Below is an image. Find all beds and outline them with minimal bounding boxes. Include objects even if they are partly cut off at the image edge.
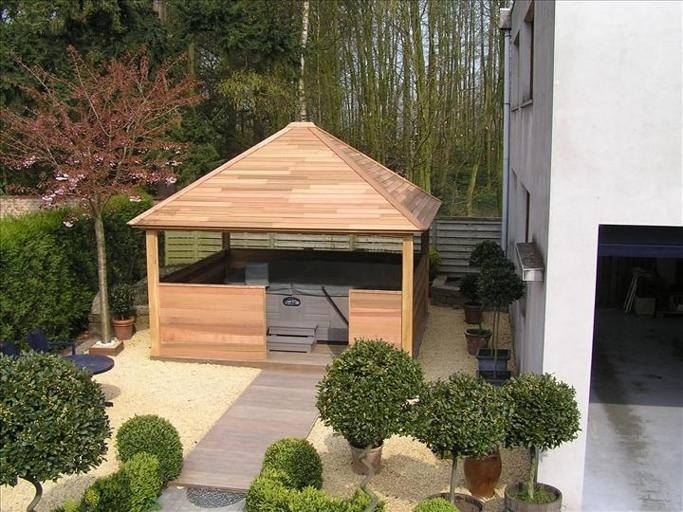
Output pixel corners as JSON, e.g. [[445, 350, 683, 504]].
[[225, 268, 361, 342]]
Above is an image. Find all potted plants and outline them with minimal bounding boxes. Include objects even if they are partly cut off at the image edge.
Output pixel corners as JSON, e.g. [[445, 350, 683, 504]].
[[405, 370, 583, 512], [107, 282, 136, 340], [316, 337, 427, 474], [458, 237, 529, 387]]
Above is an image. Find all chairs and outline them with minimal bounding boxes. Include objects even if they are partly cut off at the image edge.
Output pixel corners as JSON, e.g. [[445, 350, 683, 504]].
[[0, 341, 19, 356], [27, 328, 114, 407]]
